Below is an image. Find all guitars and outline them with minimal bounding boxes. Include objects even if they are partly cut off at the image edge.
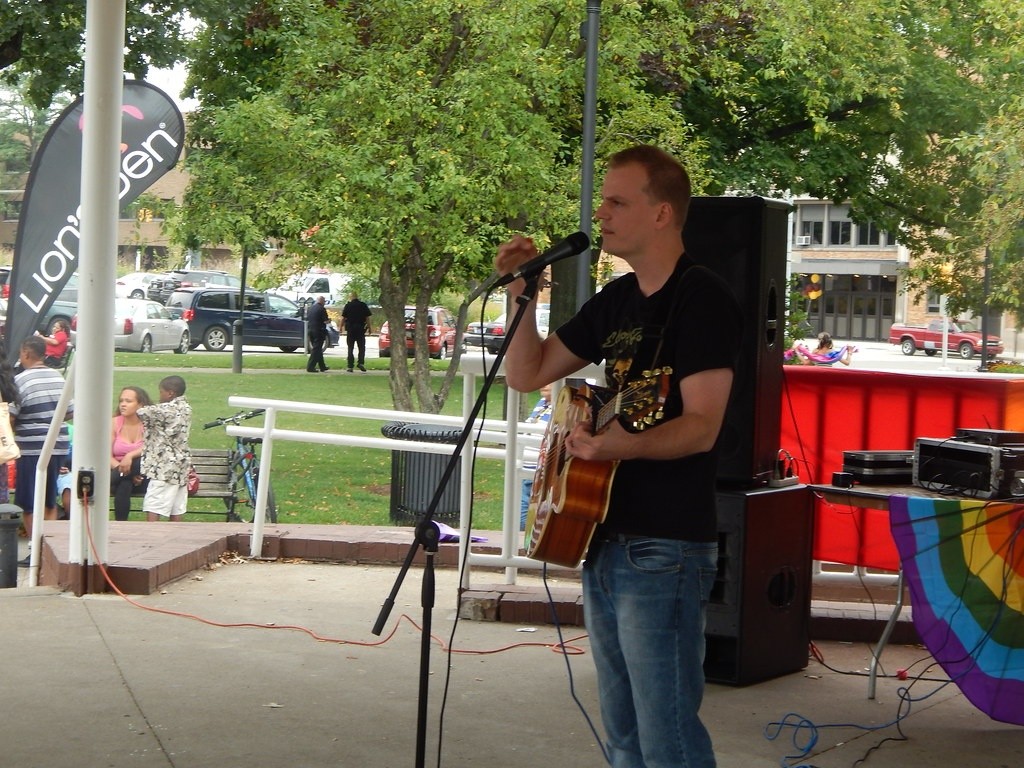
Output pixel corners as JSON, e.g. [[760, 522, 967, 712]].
[[525, 364, 675, 570]]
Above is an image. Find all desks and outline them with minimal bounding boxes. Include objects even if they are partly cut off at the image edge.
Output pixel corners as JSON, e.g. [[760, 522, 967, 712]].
[[807, 480, 1024, 699]]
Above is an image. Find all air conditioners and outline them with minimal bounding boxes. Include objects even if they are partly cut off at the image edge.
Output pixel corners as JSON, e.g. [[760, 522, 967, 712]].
[[796, 235, 810, 246]]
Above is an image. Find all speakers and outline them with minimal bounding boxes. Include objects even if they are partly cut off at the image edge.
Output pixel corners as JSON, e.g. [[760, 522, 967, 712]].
[[701, 483, 815, 689], [681, 196, 794, 491]]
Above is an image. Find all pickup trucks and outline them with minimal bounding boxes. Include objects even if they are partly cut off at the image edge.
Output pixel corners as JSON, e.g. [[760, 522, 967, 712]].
[[893, 315, 1004, 359]]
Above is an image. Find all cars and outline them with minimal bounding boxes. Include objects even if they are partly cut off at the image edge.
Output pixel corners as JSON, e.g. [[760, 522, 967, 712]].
[[166, 288, 339, 352], [467, 303, 549, 354], [116, 272, 167, 299], [69, 298, 192, 354]]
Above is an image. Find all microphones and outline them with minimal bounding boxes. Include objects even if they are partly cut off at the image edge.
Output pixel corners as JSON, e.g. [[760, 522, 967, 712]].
[[494, 232, 590, 288]]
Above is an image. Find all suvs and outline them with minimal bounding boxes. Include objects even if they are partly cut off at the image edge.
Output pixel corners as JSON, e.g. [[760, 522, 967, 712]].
[[379, 305, 467, 360], [147, 270, 257, 305], [0, 266, 78, 338]]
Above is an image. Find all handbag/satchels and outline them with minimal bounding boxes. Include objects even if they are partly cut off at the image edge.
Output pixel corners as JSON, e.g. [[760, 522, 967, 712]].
[[0, 398, 22, 465], [186, 466, 199, 493]]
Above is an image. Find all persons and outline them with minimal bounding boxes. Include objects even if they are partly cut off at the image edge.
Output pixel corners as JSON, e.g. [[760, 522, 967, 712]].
[[111, 386, 152, 520], [34, 321, 70, 366], [520, 384, 553, 532], [307, 296, 331, 372], [8, 335, 74, 567], [496, 146, 745, 768], [136, 375, 192, 522], [0, 336, 21, 500], [57, 424, 73, 519], [339, 292, 371, 372], [793, 332, 852, 367]]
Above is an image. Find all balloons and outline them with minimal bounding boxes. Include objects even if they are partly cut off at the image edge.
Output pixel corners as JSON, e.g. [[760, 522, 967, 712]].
[[801, 275, 822, 300]]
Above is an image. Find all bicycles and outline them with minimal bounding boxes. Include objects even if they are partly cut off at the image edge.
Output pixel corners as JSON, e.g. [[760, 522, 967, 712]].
[[202, 409, 277, 524]]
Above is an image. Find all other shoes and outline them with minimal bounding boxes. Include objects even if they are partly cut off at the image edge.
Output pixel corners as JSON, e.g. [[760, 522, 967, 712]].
[[308, 369, 318, 372], [347, 368, 352, 371], [357, 364, 366, 372], [322, 367, 329, 371], [17, 554, 29, 567]]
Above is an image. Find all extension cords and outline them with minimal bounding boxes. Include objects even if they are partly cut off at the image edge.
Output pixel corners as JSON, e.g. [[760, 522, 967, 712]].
[[769, 474, 799, 487]]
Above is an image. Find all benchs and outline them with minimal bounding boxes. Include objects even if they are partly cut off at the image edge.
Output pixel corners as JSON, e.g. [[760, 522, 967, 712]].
[[8, 448, 238, 521]]
